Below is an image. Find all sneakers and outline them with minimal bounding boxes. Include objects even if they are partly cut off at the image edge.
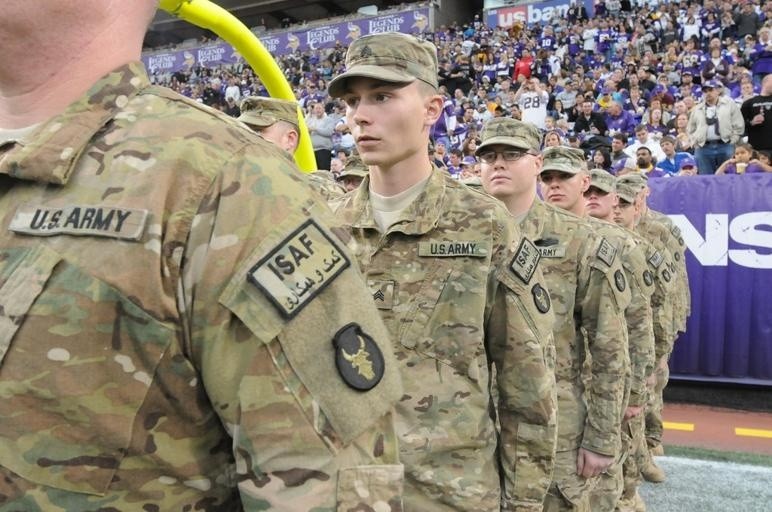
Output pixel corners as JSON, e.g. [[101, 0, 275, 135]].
[[640, 463, 665, 483], [634, 493, 646, 512], [651, 443, 666, 456]]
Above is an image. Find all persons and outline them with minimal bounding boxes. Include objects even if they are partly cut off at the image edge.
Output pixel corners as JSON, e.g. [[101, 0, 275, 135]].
[[1, 0, 772, 511]]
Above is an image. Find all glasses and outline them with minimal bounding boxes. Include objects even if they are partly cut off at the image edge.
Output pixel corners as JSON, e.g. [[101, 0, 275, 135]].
[[477, 147, 529, 164]]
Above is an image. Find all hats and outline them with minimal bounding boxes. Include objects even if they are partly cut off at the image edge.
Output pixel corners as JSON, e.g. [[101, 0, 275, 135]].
[[614, 173, 643, 192], [701, 79, 719, 91], [539, 145, 589, 179], [680, 158, 696, 170], [583, 168, 618, 193], [629, 171, 649, 186], [473, 116, 544, 158], [615, 182, 638, 204], [326, 30, 440, 99], [436, 139, 446, 145], [234, 94, 300, 130]]
